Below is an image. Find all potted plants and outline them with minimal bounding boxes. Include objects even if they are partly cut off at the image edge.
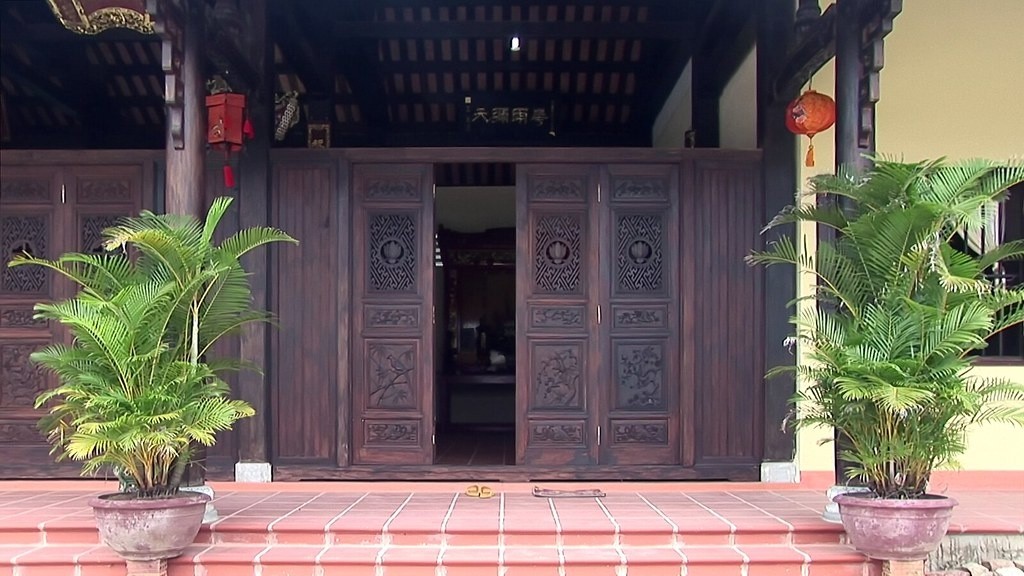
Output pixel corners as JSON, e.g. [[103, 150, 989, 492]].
[[745, 155, 1024, 562], [9, 197, 297, 560]]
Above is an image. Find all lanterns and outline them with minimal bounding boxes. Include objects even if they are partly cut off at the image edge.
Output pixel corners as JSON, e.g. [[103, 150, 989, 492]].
[[786, 86, 836, 167], [204, 95, 245, 189]]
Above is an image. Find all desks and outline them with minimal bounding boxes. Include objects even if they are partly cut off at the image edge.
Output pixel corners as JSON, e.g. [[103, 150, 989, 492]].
[[438, 372, 515, 436]]
